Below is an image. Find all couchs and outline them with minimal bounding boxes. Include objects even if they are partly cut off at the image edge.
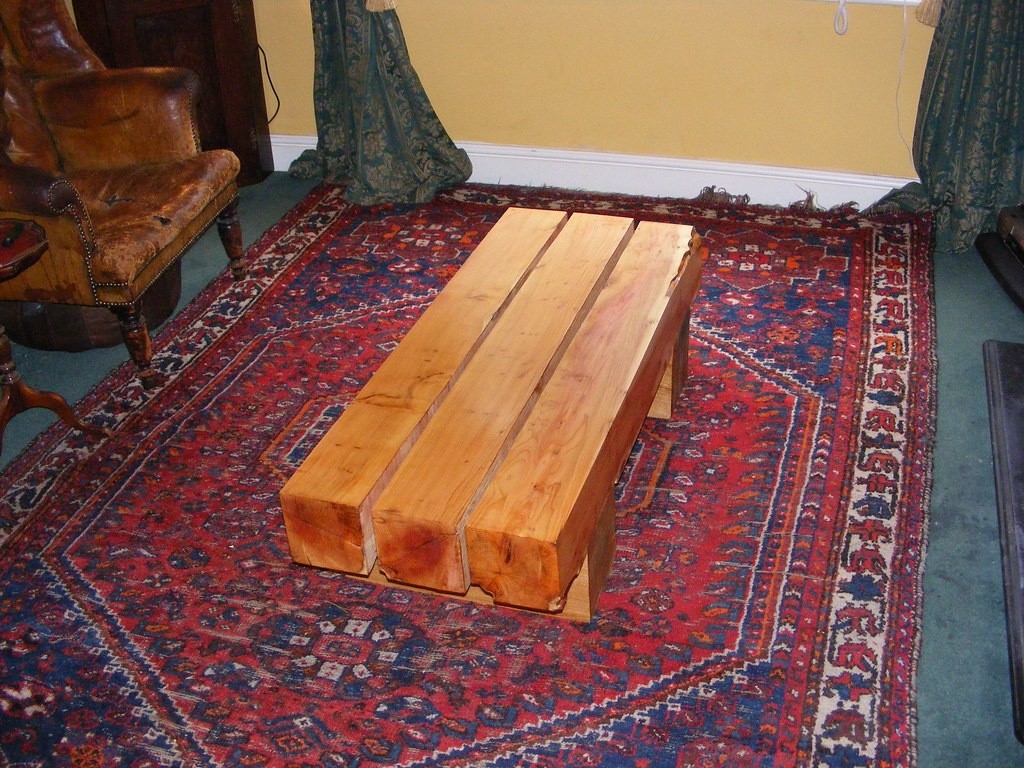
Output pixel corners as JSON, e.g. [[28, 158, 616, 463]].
[[0, 0, 247, 392]]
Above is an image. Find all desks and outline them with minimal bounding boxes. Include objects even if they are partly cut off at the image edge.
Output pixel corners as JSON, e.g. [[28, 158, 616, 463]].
[[280, 206, 703, 624], [0, 218, 115, 455]]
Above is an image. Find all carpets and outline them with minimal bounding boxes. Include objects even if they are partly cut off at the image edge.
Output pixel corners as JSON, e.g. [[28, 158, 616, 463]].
[[0, 183, 938, 767]]
